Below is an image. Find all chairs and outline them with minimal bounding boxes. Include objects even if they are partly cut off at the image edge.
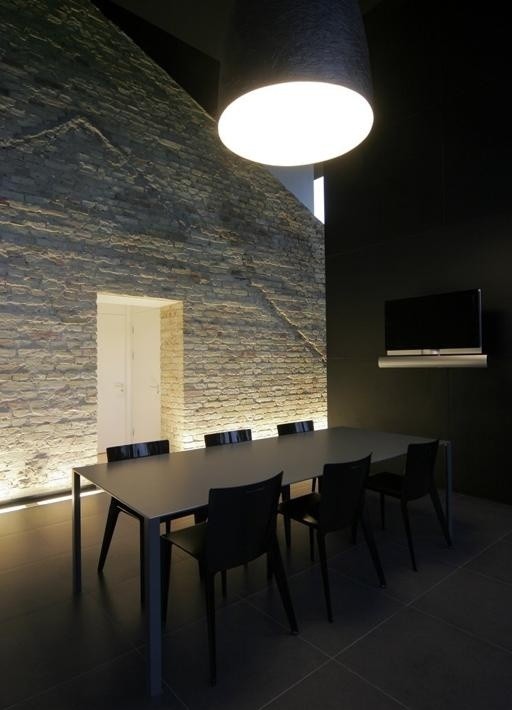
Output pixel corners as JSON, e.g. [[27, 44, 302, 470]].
[[277, 421, 316, 502], [266, 452, 388, 625], [192, 429, 251, 525], [349, 440, 455, 572], [97, 440, 171, 611], [161, 470, 298, 688]]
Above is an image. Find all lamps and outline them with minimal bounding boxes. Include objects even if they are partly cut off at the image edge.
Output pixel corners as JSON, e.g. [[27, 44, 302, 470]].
[[215, 0, 378, 167]]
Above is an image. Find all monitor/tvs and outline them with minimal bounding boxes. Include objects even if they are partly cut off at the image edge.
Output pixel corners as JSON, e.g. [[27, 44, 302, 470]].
[[385, 289, 482, 357]]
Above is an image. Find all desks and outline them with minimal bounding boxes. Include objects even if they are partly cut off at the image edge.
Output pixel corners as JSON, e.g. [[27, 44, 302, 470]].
[[71, 427, 454, 699]]
